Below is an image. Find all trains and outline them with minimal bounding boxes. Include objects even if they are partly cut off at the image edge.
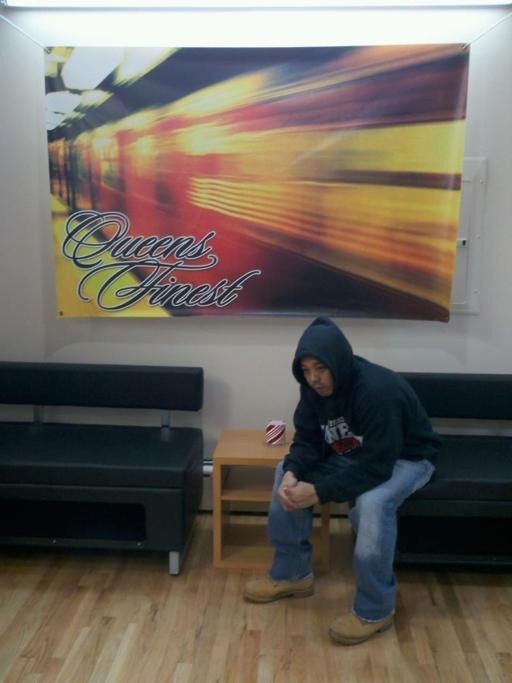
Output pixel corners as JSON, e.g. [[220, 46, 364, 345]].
[[49, 47, 466, 320]]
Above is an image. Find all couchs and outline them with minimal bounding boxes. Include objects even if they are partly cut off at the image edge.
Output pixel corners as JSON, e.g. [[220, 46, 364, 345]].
[[0, 360, 203, 575], [352, 373, 511, 573]]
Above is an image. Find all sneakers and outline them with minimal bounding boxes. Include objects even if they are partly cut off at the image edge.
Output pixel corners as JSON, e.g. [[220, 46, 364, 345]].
[[243, 571, 315, 604], [329, 606, 395, 646]]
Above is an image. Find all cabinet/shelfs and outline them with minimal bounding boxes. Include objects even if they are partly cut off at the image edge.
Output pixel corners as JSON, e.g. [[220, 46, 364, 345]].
[[212, 428, 330, 573]]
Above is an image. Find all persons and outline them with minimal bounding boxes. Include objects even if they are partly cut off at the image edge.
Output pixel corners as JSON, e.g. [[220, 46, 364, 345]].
[[242, 316, 436, 645]]
